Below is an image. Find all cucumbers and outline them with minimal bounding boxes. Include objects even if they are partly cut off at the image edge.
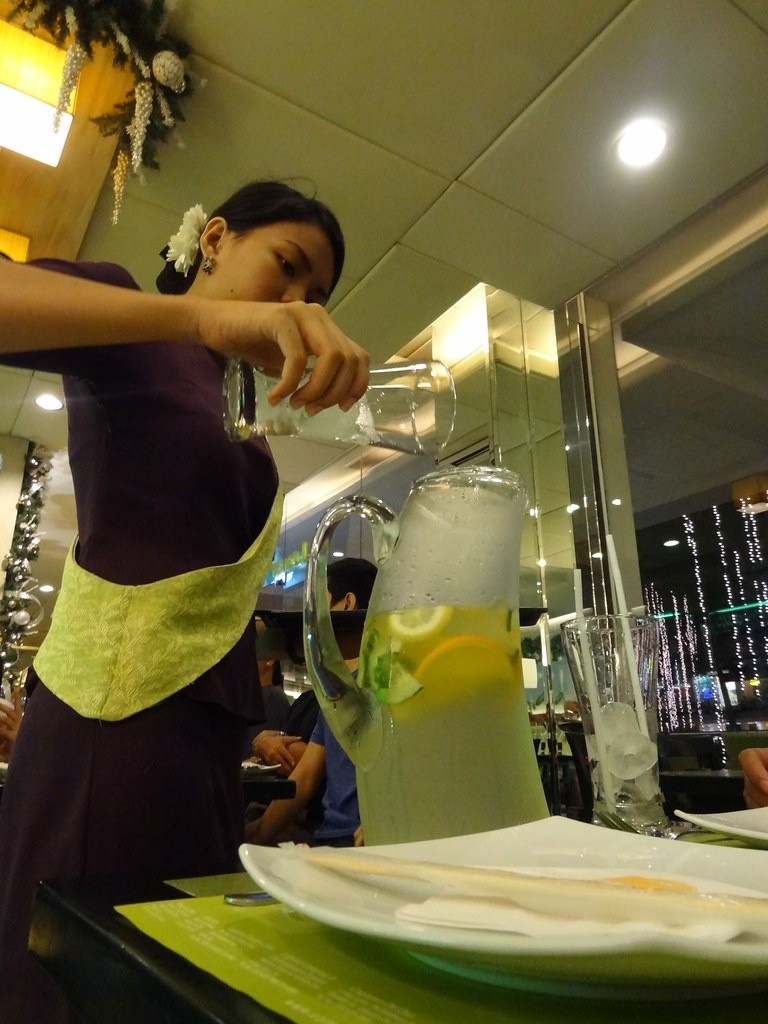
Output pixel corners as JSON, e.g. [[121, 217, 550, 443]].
[[355, 629, 423, 706]]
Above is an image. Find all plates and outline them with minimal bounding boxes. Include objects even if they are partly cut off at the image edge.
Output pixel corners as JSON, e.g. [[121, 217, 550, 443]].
[[242, 762, 282, 775], [239, 815, 767, 1004], [674, 807, 768, 848]]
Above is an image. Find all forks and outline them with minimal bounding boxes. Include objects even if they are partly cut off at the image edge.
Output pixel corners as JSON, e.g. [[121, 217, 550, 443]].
[[594, 811, 715, 841]]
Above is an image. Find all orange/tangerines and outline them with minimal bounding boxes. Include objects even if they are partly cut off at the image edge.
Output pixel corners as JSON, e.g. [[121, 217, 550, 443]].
[[415, 635, 512, 701]]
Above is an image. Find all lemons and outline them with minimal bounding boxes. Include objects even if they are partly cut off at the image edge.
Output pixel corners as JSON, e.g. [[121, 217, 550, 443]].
[[388, 604, 453, 640]]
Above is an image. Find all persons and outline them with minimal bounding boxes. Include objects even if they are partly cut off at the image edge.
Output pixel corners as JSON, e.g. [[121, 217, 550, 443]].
[[0, 177, 372, 1022], [244, 557, 378, 847], [738, 747, 768, 810]]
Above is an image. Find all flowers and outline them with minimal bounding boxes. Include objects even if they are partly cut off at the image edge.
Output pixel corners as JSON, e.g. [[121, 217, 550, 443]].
[[165, 203, 207, 279]]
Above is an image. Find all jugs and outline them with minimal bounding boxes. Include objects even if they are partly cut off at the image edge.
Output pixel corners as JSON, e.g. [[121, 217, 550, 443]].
[[303, 466, 550, 846]]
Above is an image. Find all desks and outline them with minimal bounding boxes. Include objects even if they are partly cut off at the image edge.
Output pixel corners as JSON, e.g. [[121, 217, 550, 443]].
[[35, 812, 768, 1024]]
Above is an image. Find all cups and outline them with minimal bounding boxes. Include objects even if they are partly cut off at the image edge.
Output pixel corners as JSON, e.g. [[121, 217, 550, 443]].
[[222, 354, 457, 456], [560, 615, 673, 841]]
